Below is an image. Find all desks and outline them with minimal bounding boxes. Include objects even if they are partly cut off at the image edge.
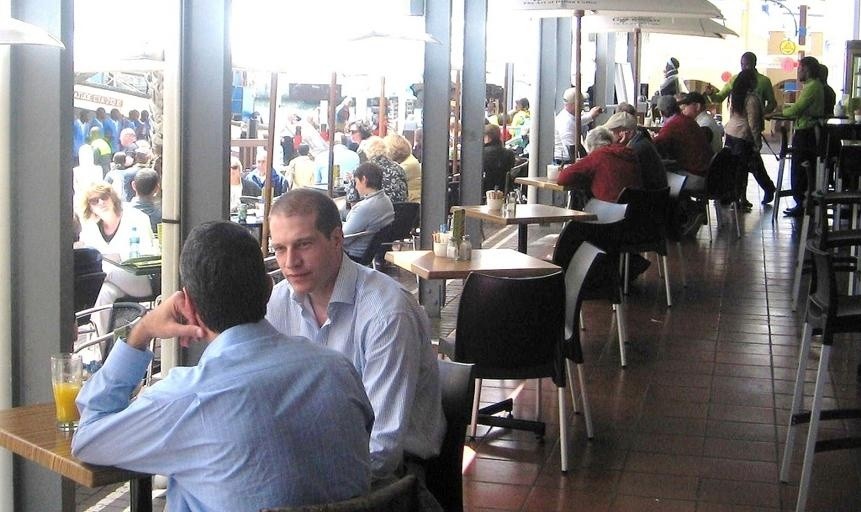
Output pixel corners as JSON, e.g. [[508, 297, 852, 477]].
[[515, 178, 573, 263]]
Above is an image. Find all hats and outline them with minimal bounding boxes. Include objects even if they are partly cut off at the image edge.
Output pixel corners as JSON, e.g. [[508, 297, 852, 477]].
[[563, 88, 590, 103], [653, 95, 676, 110], [676, 92, 705, 106], [602, 112, 637, 129]]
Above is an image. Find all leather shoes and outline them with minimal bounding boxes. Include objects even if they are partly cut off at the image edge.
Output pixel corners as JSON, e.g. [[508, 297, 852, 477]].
[[740, 187, 802, 215]]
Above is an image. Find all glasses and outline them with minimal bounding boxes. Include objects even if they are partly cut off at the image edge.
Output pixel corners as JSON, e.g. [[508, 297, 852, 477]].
[[88, 191, 110, 205]]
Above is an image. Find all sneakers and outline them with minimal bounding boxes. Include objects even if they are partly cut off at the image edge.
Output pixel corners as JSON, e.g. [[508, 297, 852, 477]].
[[629, 256, 651, 280]]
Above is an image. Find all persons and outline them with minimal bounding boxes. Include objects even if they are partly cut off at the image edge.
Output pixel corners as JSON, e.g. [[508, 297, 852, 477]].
[[70, 217, 375, 512], [264, 186, 450, 483], [67, 96, 534, 366], [552, 50, 838, 289]]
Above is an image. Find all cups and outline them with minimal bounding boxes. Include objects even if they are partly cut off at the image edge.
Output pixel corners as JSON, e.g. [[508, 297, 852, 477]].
[[547, 165, 558, 180], [238, 204, 248, 221], [50, 352, 83, 431], [336, 178, 344, 187], [255, 203, 264, 217], [487, 199, 503, 210], [644, 118, 651, 127], [433, 242, 447, 257], [834, 105, 846, 118]]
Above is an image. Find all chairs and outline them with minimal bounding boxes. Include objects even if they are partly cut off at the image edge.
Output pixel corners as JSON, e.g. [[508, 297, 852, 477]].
[[445, 182, 462, 228], [686, 149, 742, 245], [581, 198, 630, 223], [619, 171, 687, 307], [768, 112, 861, 512], [502, 158, 529, 197], [714, 143, 746, 240]]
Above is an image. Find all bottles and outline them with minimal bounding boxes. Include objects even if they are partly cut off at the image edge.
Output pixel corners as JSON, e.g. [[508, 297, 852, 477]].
[[854, 109, 861, 122], [655, 118, 660, 127], [459, 235, 472, 260], [509, 192, 517, 210], [128, 227, 140, 258], [447, 237, 459, 260]]
